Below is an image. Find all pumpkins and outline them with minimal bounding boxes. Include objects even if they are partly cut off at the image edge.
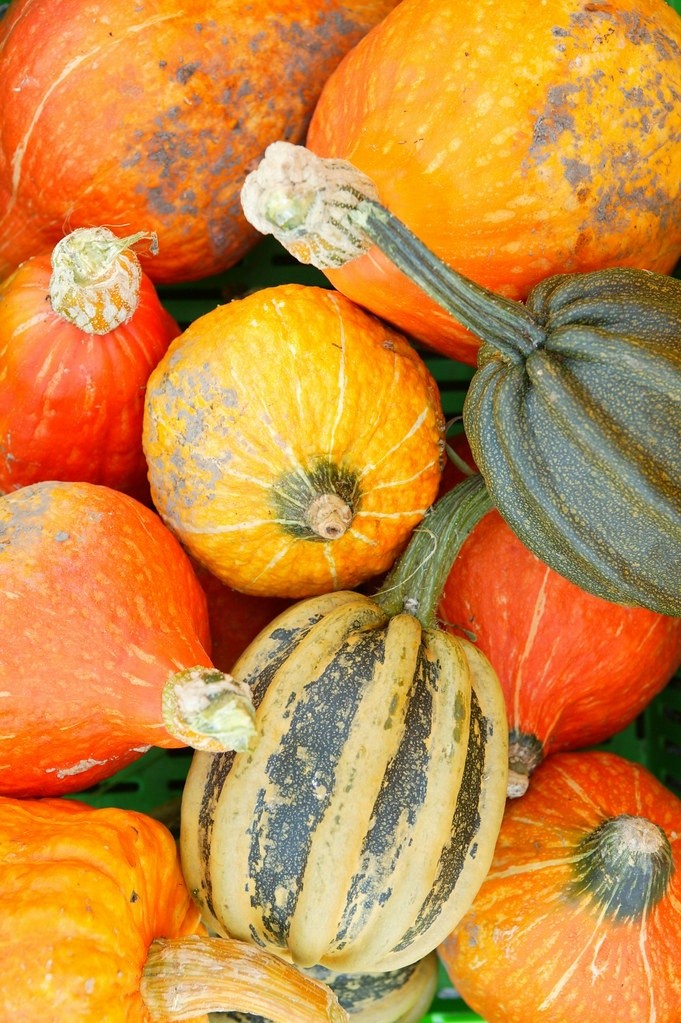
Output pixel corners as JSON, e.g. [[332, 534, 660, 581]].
[[0, 0, 681, 1023]]
